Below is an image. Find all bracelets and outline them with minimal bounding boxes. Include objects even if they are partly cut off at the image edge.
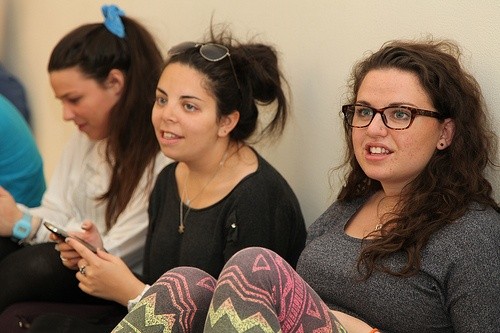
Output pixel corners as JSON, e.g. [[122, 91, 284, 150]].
[[369, 328, 378, 333]]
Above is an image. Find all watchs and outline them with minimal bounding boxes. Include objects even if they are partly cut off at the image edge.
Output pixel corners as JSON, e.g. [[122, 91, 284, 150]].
[[12, 213, 33, 242]]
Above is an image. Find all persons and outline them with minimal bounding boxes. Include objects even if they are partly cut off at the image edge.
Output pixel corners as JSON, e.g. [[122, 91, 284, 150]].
[[0, 5, 305, 333], [109, 40, 500, 333]]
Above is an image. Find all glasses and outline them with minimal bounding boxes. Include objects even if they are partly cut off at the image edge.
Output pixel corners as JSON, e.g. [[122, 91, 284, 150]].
[[167, 40, 240, 87], [342, 103, 443, 130]]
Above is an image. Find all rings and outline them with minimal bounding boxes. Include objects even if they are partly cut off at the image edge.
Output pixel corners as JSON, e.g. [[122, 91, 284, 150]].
[[78, 265, 86, 274]]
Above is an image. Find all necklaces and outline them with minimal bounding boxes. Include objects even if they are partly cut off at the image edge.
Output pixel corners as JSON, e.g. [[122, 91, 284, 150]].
[[177, 151, 229, 235], [374, 192, 400, 231]]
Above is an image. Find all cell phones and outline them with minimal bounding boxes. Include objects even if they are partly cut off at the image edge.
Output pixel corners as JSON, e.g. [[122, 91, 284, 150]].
[[44, 222, 98, 255]]
